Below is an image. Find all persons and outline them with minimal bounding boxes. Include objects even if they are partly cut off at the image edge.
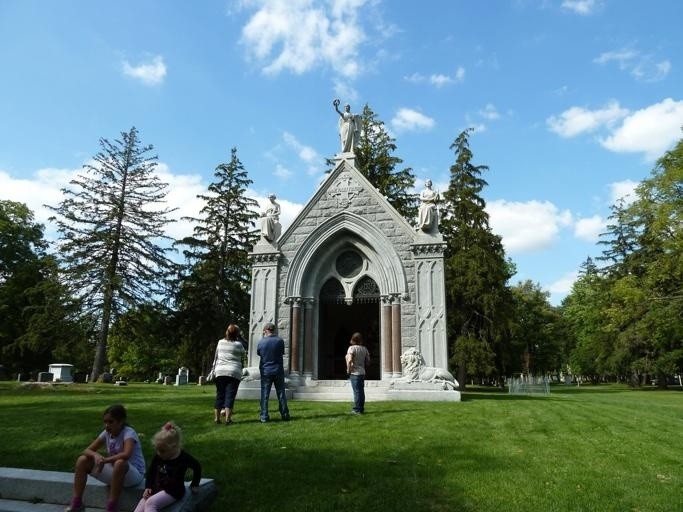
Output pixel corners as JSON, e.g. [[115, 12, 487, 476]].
[[332, 98, 363, 154], [63, 402, 145, 510], [416, 179, 441, 231], [255, 323, 290, 423], [211, 324, 247, 424], [259, 192, 282, 240], [128, 419, 201, 512], [343, 331, 373, 415]]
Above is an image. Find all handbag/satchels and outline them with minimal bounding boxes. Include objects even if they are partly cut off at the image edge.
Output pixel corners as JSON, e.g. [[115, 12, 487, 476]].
[[205, 372, 215, 381]]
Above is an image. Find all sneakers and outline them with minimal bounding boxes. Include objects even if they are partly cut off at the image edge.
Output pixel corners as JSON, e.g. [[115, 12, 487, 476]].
[[213, 409, 289, 426], [351, 409, 364, 416]]
